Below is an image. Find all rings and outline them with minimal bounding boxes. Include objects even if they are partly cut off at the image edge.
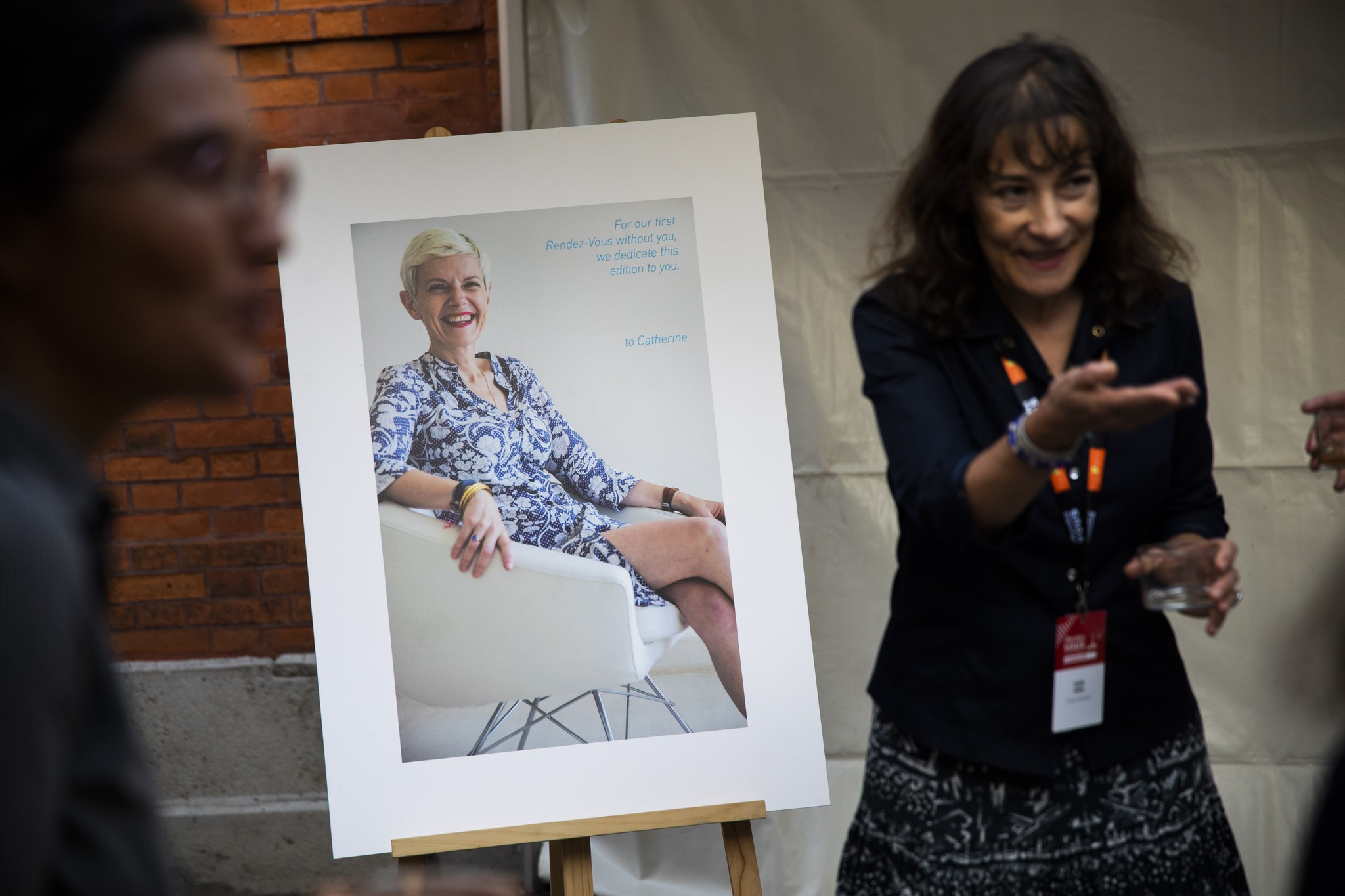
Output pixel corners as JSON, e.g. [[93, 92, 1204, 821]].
[[470, 535, 482, 542]]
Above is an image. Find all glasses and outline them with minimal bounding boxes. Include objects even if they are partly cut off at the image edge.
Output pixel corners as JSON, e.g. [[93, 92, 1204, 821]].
[[37, 131, 294, 218]]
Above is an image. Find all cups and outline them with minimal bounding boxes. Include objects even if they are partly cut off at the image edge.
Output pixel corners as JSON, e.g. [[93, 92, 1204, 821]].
[[1137, 540, 1218, 608]]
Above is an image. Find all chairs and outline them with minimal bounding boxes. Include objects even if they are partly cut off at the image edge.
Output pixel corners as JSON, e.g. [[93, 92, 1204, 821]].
[[379, 500, 691, 756]]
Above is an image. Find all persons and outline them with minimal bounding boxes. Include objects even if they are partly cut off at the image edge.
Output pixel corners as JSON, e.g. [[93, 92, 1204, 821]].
[[1291, 392, 1345, 896], [368, 228, 749, 722], [2, 0, 300, 896], [825, 40, 1254, 896]]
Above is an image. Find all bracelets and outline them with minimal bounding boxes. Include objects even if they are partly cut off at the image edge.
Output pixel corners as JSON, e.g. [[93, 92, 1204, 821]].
[[1007, 416, 1080, 468], [450, 478, 493, 519], [661, 487, 679, 512]]
